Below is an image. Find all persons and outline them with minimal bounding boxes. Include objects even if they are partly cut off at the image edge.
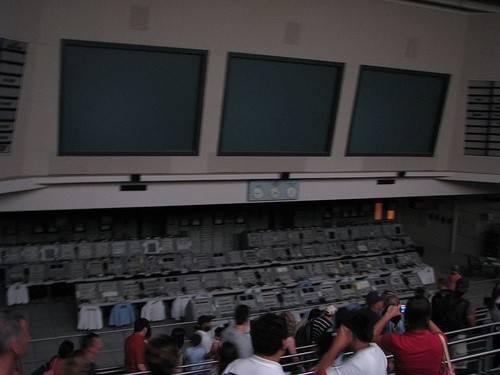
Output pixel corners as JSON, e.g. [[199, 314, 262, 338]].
[[0, 264, 500, 375]]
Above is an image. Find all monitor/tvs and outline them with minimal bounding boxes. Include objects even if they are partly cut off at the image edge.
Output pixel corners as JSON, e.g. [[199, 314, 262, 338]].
[[58, 38, 452, 157]]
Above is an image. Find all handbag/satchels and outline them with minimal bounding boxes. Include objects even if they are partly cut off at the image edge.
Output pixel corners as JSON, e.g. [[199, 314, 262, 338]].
[[437, 333, 455, 375]]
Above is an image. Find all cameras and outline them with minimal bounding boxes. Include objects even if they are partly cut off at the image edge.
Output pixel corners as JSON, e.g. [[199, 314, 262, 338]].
[[400, 305, 406, 313]]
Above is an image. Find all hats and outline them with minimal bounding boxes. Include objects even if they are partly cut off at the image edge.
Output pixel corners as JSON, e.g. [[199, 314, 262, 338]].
[[325, 305, 338, 316], [199, 314, 215, 326]]
